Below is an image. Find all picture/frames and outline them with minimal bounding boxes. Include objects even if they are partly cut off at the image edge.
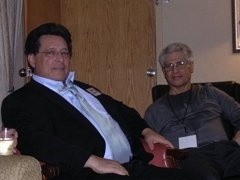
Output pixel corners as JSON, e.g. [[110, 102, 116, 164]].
[[231, 0, 240, 53]]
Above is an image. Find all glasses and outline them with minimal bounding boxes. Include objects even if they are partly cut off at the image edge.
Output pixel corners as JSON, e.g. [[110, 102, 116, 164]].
[[38, 49, 70, 58], [163, 60, 190, 70]]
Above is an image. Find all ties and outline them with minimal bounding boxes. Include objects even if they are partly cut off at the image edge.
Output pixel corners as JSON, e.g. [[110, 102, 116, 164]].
[[61, 84, 129, 164]]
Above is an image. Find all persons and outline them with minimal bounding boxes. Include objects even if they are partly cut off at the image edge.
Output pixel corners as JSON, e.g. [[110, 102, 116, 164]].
[[144, 43, 240, 180], [0, 22, 207, 180]]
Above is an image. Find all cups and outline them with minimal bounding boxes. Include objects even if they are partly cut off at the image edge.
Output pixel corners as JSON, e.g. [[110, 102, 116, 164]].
[[1, 127, 16, 156]]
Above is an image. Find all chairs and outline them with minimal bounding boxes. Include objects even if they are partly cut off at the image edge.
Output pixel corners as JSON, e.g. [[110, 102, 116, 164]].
[[142, 81, 240, 168]]
